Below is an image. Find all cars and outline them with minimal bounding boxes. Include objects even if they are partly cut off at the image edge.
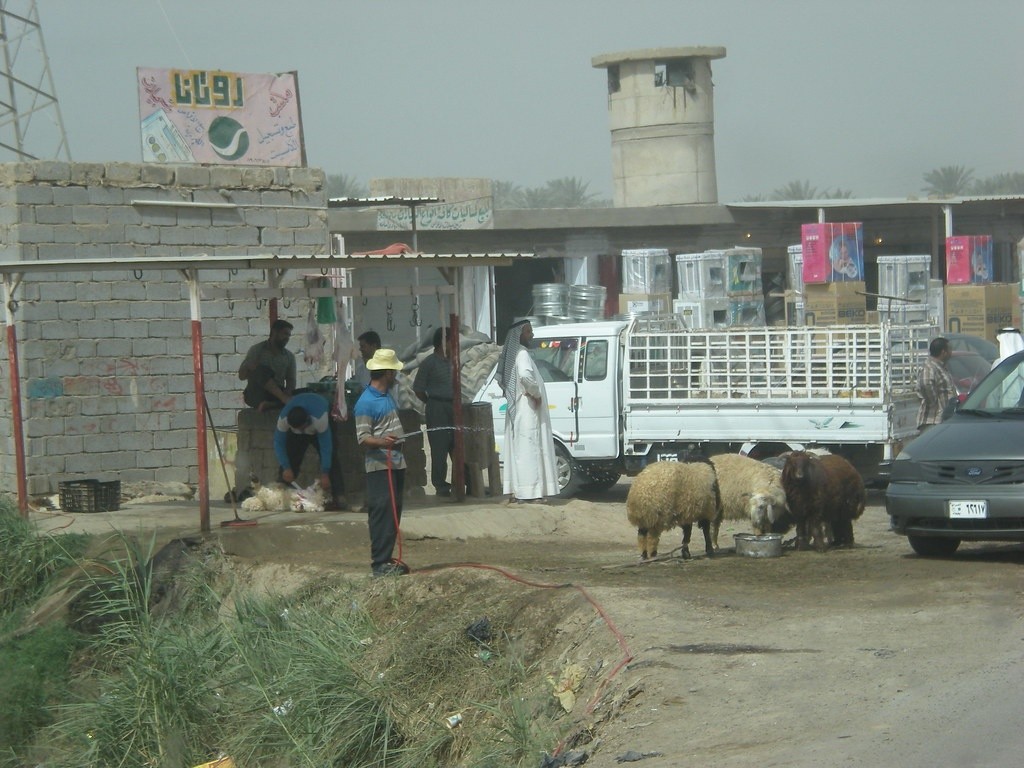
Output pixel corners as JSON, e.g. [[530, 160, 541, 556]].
[[796, 335, 998, 392], [884, 351, 1024, 558]]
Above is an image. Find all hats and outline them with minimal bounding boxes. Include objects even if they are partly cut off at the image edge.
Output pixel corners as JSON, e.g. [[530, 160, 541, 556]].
[[366, 348, 403, 370]]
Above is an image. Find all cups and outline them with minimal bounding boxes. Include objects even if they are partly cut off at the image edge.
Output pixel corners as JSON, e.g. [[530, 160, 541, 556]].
[[445, 713, 463, 730]]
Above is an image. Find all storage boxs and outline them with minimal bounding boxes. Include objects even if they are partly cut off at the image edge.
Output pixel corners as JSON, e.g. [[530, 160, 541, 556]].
[[802, 221, 864, 284], [58, 479, 121, 513], [618, 292, 672, 318], [943, 284, 1013, 353], [804, 282, 865, 353], [946, 236, 993, 284]]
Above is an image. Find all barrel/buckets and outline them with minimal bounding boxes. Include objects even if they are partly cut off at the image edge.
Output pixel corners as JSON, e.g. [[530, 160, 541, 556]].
[[512, 282, 609, 328]]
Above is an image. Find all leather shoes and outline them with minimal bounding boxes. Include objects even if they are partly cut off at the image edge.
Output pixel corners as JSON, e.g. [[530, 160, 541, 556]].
[[372, 562, 407, 578]]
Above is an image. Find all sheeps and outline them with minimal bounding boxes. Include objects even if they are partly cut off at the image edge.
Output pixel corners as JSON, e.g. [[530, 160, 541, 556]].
[[709, 454, 794, 553], [777, 450, 867, 554], [624, 450, 722, 564]]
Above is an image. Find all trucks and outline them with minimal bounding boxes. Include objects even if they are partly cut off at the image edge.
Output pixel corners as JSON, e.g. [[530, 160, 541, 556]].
[[474, 312, 939, 502]]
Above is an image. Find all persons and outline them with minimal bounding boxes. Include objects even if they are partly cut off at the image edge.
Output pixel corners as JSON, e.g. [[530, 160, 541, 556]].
[[239, 320, 296, 408], [985, 327, 1024, 409], [835, 240, 853, 270], [977, 249, 986, 273], [915, 337, 958, 434], [273, 393, 348, 510], [495, 318, 560, 504], [413, 327, 490, 498], [354, 331, 411, 576]]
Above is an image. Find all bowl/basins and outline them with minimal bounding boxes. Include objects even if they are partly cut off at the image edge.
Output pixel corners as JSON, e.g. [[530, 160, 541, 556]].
[[733, 531, 786, 559]]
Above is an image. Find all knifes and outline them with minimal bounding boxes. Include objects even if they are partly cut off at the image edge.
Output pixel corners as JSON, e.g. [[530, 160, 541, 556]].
[[290, 480, 305, 490]]
